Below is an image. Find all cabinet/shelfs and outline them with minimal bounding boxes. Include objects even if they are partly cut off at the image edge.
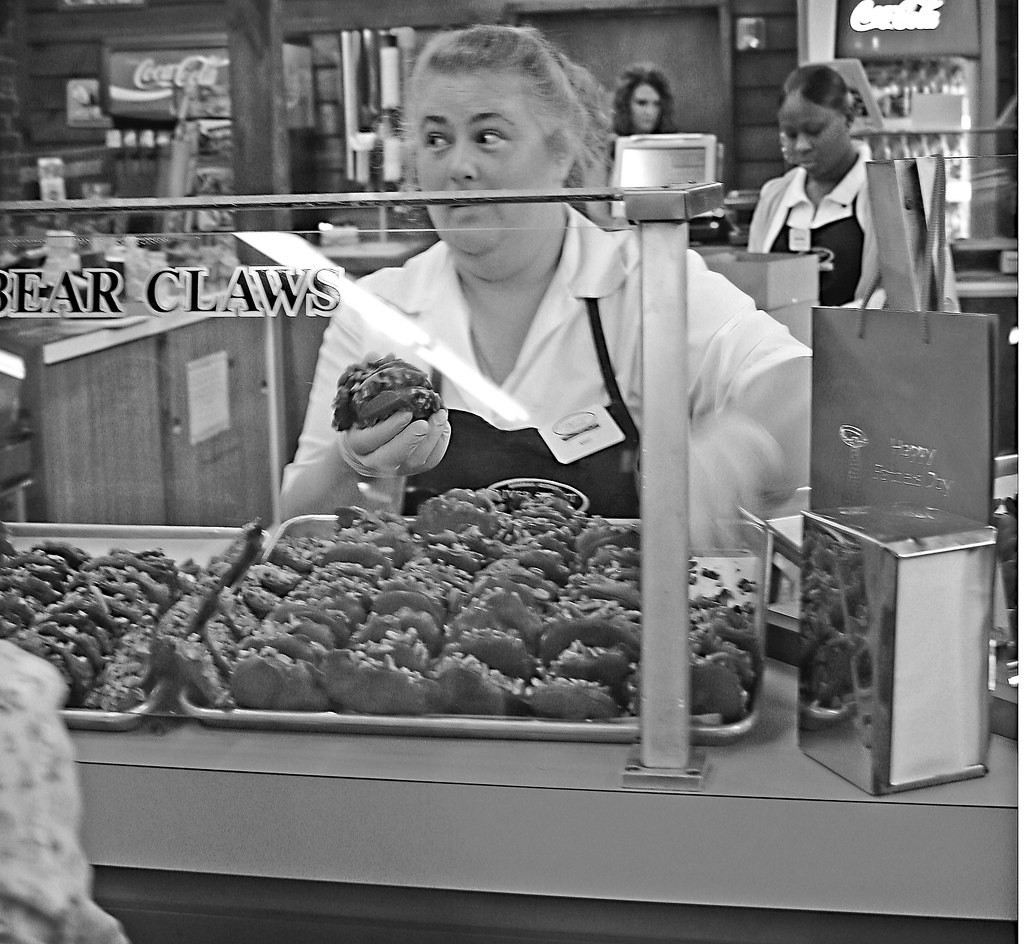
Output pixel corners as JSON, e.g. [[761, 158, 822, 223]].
[[0, 305, 332, 530]]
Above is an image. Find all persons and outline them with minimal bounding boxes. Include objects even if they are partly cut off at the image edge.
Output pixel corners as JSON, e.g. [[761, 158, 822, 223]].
[[747, 67, 893, 311], [276, 23, 813, 554], [579, 61, 678, 231]]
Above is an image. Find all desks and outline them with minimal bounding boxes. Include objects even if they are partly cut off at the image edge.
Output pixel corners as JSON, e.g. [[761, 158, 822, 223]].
[[320, 240, 431, 269]]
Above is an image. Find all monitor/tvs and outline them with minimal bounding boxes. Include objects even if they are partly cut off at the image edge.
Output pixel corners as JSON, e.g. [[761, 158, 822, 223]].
[[610, 132, 717, 217]]
